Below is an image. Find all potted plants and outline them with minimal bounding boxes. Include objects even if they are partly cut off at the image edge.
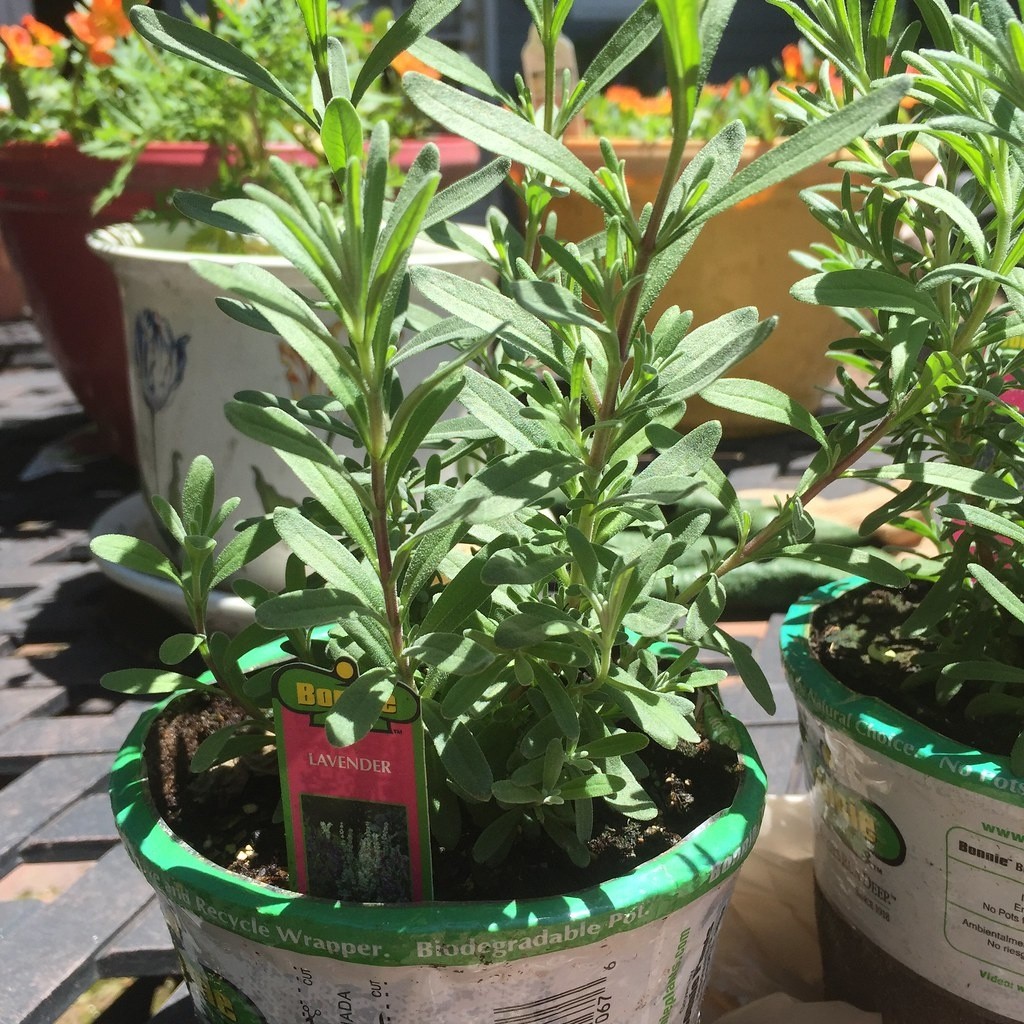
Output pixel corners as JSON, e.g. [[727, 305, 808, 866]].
[[87, 1, 1023, 1022]]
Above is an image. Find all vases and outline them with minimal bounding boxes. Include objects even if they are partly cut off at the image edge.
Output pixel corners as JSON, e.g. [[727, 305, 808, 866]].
[[509, 138, 945, 442], [1, 126, 479, 481]]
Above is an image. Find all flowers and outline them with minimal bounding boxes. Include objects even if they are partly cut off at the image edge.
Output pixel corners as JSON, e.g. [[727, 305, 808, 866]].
[[0, 0, 448, 143], [558, 45, 928, 139]]
